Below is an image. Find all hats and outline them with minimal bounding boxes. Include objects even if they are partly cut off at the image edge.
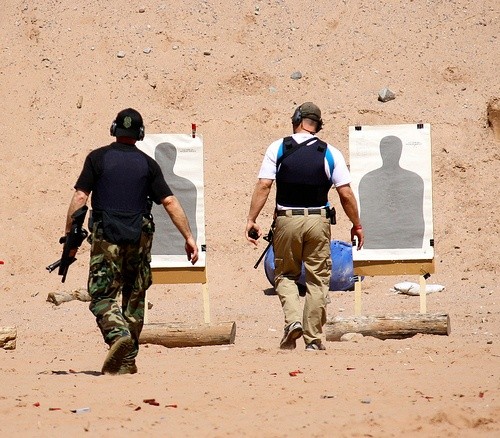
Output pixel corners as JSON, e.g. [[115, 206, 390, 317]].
[[292, 101, 322, 121], [112, 107, 144, 139]]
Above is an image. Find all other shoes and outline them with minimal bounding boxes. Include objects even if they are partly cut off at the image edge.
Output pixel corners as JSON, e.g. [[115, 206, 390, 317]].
[[279, 322, 304, 350], [119, 364, 139, 374], [101, 335, 134, 376], [305, 340, 329, 352]]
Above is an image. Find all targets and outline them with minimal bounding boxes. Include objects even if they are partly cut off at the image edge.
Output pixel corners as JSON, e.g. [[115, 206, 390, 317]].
[[133, 132, 206, 268], [348, 121, 434, 261]]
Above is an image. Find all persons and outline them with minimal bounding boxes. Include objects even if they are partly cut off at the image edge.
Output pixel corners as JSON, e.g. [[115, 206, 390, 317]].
[[245, 101, 364, 352], [64, 107, 198, 375]]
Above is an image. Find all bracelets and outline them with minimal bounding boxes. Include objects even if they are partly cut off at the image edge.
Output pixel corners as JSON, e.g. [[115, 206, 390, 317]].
[[354, 225, 362, 229]]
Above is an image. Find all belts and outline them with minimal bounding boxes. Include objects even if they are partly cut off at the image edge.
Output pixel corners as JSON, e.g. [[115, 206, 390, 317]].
[[277, 209, 323, 217]]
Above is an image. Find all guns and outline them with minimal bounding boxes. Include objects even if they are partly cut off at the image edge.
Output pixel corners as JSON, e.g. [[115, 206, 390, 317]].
[[254, 220, 276, 269], [46, 205, 89, 283]]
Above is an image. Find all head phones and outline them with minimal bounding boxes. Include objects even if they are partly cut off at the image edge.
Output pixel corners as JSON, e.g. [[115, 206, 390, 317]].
[[110, 120, 145, 141], [291, 106, 324, 133]]
